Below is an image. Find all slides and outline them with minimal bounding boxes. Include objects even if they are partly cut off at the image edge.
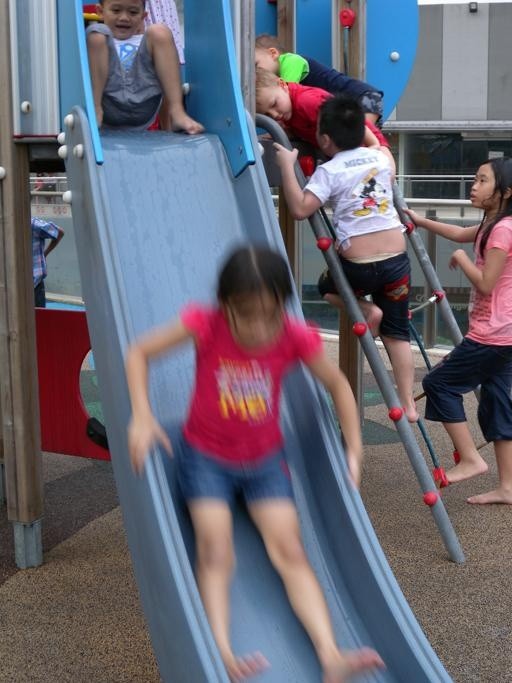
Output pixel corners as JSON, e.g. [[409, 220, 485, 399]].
[[59, 106, 450, 677]]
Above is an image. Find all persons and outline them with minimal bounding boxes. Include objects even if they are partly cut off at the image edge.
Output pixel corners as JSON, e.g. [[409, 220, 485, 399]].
[[31, 216, 66, 309], [253, 68, 389, 172], [273, 99, 418, 423], [83, 1, 204, 134], [253, 37, 383, 129], [402, 157, 512, 506], [126, 245, 390, 682]]
